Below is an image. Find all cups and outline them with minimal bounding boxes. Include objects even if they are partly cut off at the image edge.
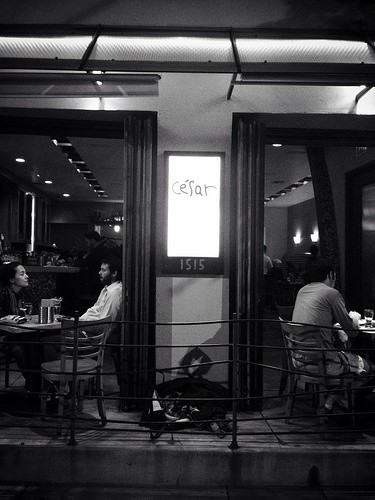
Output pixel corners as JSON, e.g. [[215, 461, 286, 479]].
[[39, 306, 54, 323], [18, 302, 33, 320], [364, 309, 374, 327]]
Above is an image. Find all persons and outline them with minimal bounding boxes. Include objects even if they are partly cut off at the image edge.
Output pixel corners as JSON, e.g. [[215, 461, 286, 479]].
[[290, 260, 370, 412], [262, 245, 274, 306], [305, 244, 321, 283], [0, 261, 58, 397], [82, 232, 110, 295], [44, 259, 124, 402]]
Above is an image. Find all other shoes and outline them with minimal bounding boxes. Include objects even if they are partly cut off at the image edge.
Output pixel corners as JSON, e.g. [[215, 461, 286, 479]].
[[333, 397, 353, 417], [317, 406, 339, 430]]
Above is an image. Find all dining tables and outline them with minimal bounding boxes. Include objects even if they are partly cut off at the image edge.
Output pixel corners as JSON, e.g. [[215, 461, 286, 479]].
[[278, 279, 303, 306], [358, 318, 375, 342], [0, 315, 76, 414]]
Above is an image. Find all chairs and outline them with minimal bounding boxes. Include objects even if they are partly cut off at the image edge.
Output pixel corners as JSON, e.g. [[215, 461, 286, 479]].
[[78, 293, 98, 316], [40, 315, 113, 437], [278, 317, 358, 443], [278, 343, 315, 400], [0, 352, 22, 388]]
